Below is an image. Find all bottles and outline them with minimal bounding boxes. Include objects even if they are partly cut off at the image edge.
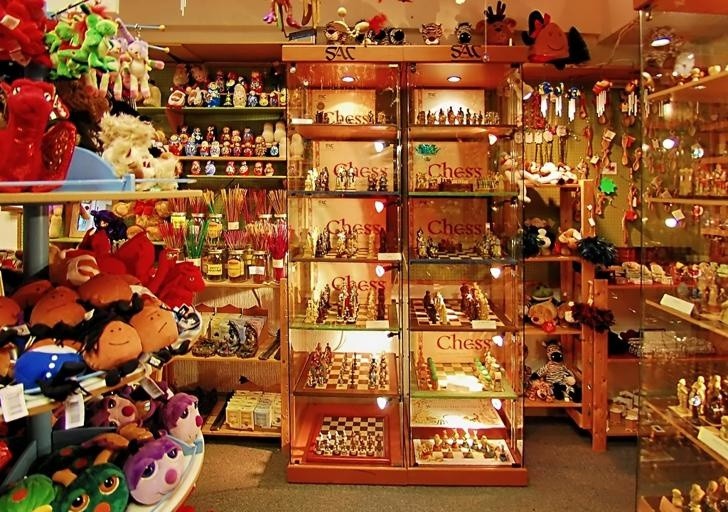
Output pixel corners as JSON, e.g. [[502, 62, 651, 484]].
[[161, 208, 287, 285]]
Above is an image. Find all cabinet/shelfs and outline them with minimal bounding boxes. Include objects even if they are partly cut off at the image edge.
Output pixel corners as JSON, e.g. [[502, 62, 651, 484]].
[[0, 178, 205, 512]]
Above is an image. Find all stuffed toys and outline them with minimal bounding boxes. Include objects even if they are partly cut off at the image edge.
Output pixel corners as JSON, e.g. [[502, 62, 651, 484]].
[[526, 377, 555, 402], [524, 217, 583, 257], [530, 340, 577, 400], [0, 199, 206, 512], [0, 0, 181, 184], [1, 199, 206, 512], [325, 3, 589, 71], [524, 283, 615, 333], [501, 152, 578, 204]]
